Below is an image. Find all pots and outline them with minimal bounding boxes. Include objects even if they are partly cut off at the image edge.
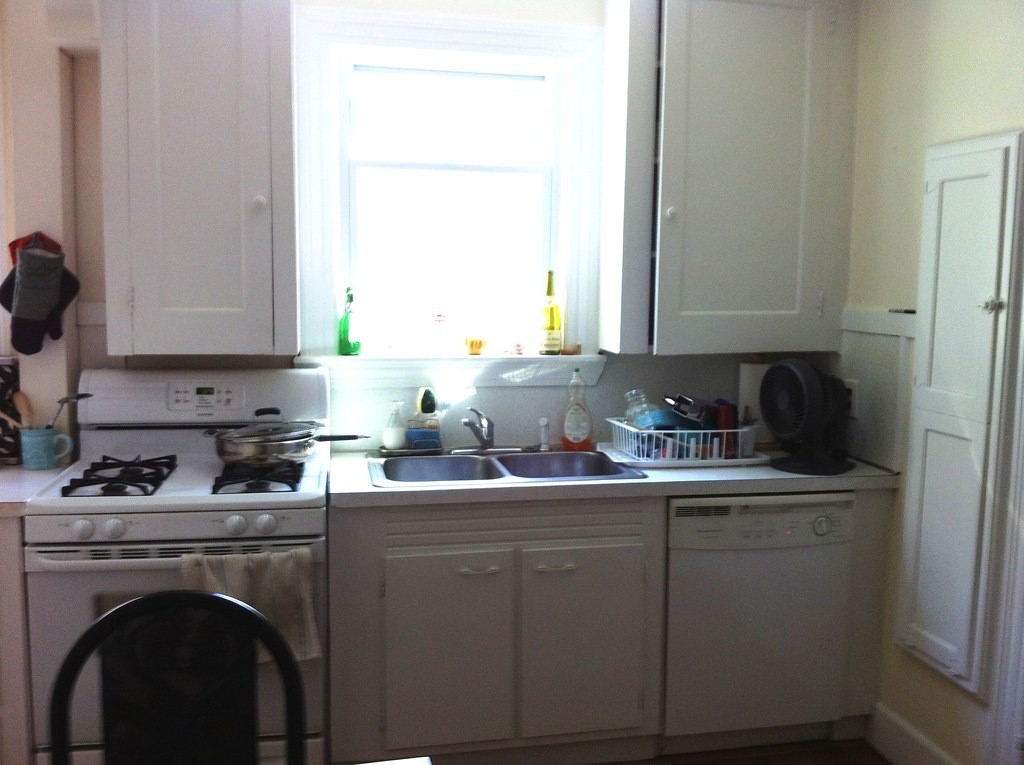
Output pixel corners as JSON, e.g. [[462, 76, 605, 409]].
[[202, 407, 371, 467]]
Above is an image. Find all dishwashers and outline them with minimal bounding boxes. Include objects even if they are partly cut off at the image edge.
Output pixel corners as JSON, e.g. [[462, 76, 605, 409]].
[[20, 542, 328, 765]]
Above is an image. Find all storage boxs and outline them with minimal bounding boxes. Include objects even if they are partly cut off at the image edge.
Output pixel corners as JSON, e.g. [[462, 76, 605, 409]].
[[606, 416, 749, 462]]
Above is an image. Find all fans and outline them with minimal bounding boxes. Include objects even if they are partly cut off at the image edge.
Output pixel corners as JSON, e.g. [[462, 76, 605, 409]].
[[759, 356, 857, 475]]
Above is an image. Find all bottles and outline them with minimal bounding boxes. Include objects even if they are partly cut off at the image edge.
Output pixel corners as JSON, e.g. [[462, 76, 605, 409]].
[[625, 387, 659, 457], [337, 285, 361, 355], [561, 368, 592, 451], [539, 268, 564, 357]]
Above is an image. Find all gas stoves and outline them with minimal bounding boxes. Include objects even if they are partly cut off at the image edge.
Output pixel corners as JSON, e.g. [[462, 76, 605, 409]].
[[22, 368, 329, 544]]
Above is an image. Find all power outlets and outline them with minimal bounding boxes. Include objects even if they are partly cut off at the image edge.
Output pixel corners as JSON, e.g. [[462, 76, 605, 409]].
[[844, 378, 859, 420]]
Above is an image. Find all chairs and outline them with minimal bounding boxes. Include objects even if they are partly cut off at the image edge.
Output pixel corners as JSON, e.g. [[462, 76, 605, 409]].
[[49, 588, 305, 765]]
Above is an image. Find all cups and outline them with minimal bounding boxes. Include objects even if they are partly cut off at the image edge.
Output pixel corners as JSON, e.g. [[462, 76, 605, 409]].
[[739, 425, 756, 456], [20, 428, 72, 470]]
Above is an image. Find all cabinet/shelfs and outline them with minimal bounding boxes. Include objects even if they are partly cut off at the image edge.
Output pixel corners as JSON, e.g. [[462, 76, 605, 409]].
[[328, 494, 667, 765], [597, 1, 858, 356], [893, 128, 1024, 710], [100, 1, 299, 357]]
